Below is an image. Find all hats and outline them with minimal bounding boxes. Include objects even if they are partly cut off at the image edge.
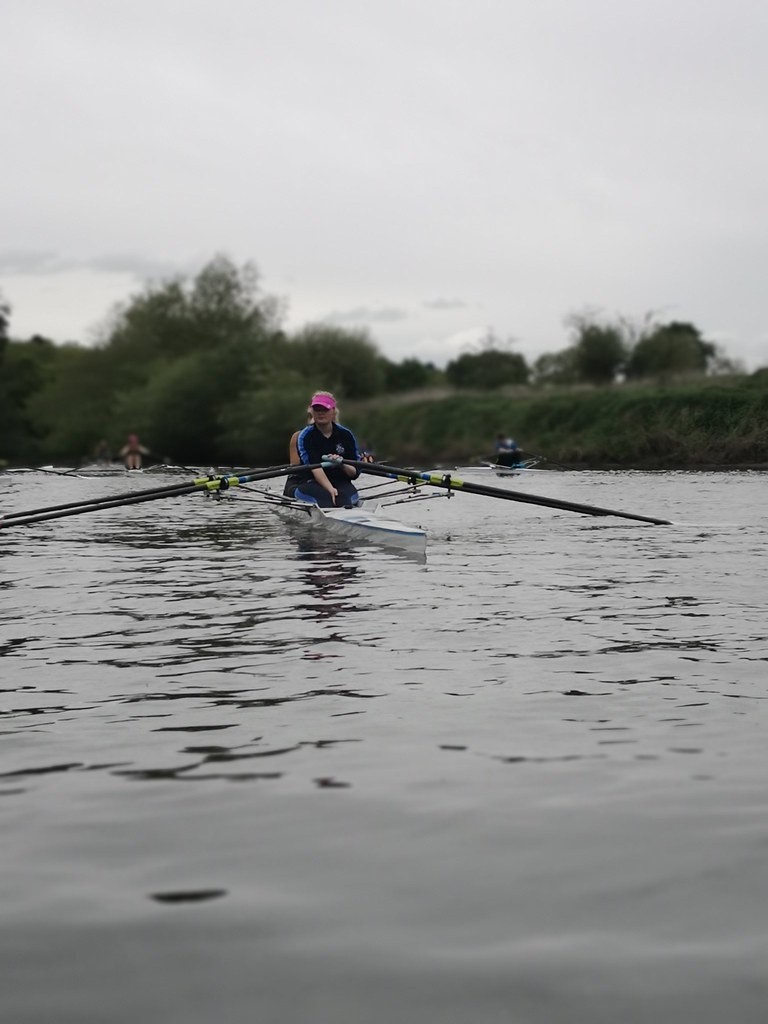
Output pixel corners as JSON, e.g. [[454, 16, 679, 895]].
[[310, 395, 336, 409]]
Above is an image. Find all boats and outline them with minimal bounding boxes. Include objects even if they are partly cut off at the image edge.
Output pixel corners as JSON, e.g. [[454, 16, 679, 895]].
[[263, 476, 427, 557]]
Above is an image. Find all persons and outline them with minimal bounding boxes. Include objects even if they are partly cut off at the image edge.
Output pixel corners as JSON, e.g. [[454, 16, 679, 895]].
[[117, 432, 152, 470], [474, 430, 551, 479], [282, 389, 375, 510]]
